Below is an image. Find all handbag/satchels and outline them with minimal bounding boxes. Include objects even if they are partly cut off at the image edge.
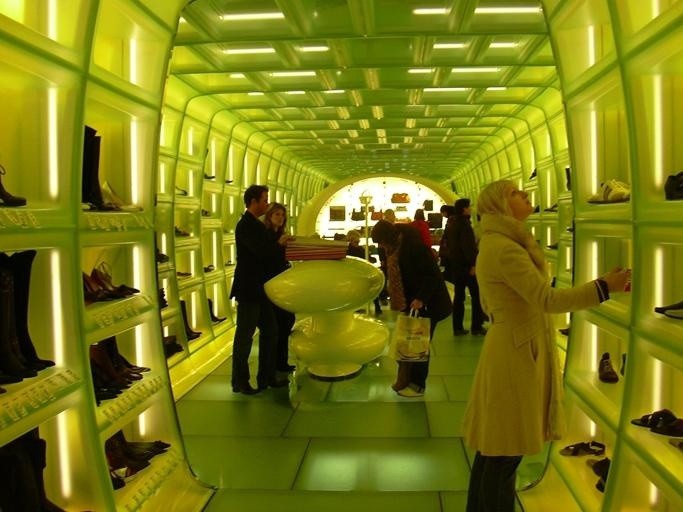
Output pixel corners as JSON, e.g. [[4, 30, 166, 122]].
[[395, 206, 408, 212], [423, 199, 434, 211], [391, 191, 411, 204], [429, 229, 446, 246], [360, 205, 375, 213], [351, 208, 365, 222], [393, 216, 412, 224], [358, 189, 373, 205], [371, 208, 384, 220], [427, 212, 443, 229], [388, 304, 432, 363], [330, 206, 345, 221], [333, 233, 346, 241], [353, 225, 366, 238]]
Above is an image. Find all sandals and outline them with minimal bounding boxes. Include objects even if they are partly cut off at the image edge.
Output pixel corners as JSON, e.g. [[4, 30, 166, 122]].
[[559, 439, 607, 458], [650, 418, 683, 437], [630, 408, 678, 430]]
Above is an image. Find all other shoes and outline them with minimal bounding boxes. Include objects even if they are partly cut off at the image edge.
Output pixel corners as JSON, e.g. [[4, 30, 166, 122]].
[[0, 166, 28, 208], [104, 429, 171, 489]]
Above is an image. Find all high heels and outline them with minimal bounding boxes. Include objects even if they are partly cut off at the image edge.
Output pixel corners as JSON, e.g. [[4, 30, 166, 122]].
[[101, 182, 141, 212], [82, 268, 141, 301]]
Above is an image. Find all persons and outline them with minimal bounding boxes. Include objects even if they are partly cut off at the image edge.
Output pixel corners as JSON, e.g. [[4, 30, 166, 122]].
[[463, 179, 631, 512], [261, 202, 288, 371], [334, 198, 489, 398], [228, 184, 296, 396]]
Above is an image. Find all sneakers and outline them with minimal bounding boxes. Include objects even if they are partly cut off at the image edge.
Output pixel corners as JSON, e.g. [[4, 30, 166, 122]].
[[598, 351, 619, 385], [664, 171, 682, 200], [471, 326, 488, 336], [391, 377, 411, 392], [398, 384, 425, 397], [453, 328, 470, 336]]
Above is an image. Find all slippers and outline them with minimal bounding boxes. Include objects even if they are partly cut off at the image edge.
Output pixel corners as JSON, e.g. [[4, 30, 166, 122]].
[[586, 457, 614, 493], [586, 177, 631, 203]]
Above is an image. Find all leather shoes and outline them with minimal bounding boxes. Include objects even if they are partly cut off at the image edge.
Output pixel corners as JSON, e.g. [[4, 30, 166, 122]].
[[521, 167, 573, 338], [232, 381, 263, 395], [255, 363, 297, 390], [175, 167, 236, 282], [653, 300, 683, 320]]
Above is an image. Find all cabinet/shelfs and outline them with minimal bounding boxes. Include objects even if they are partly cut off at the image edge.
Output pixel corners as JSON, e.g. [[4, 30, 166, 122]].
[[0, 1, 332, 512], [448, 0, 682, 512]]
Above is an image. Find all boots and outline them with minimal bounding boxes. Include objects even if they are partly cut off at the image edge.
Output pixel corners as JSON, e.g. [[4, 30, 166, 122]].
[[90, 335, 152, 406], [1, 427, 65, 512], [1, 250, 54, 384], [180, 300, 204, 341], [81, 124, 119, 212], [208, 298, 227, 323]]
